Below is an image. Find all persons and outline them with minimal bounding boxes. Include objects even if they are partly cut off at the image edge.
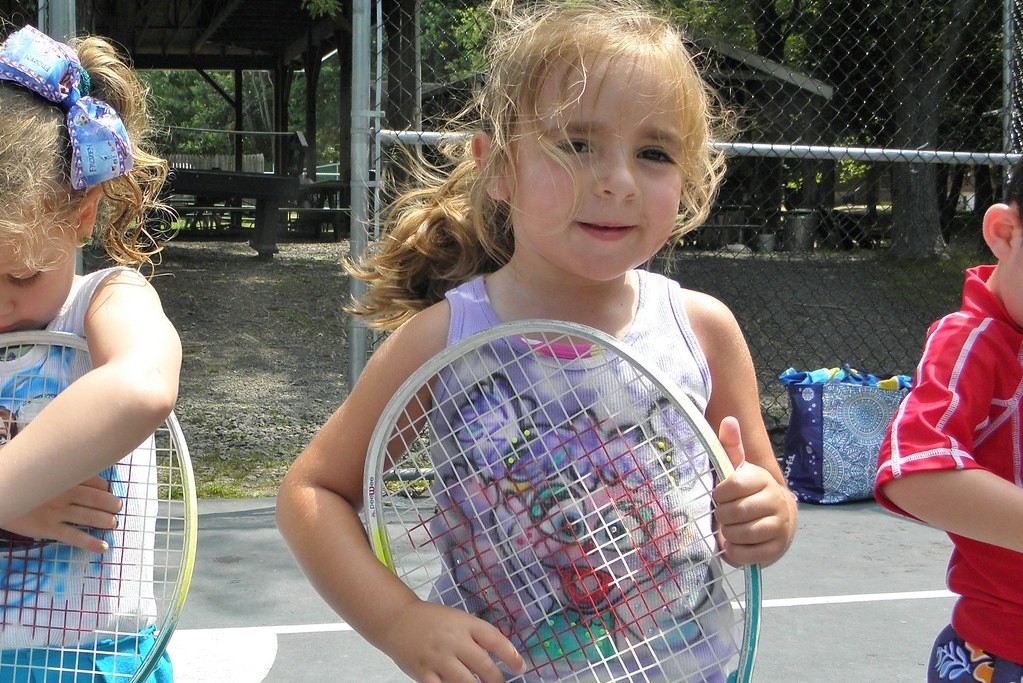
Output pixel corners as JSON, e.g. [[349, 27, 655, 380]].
[[272, 2, 805, 683], [0, 22, 187, 683], [879, 151, 1023, 683]]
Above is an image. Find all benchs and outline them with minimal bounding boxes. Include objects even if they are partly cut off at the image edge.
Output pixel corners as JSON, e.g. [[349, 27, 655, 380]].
[[142, 168, 353, 263]]
